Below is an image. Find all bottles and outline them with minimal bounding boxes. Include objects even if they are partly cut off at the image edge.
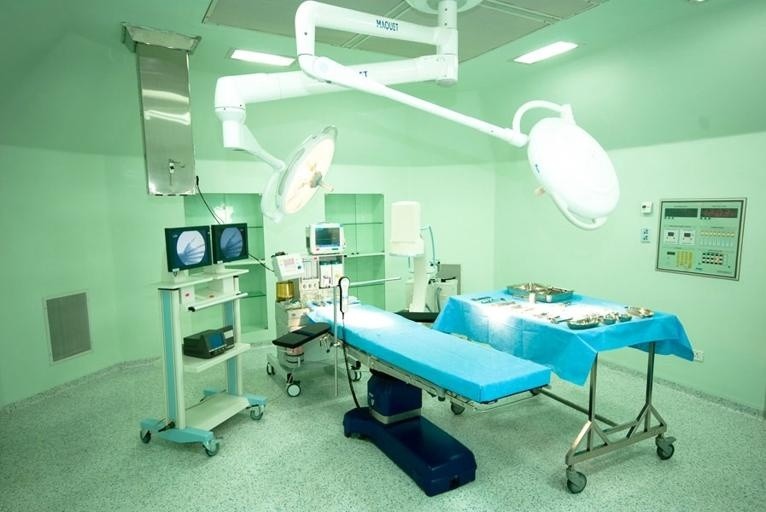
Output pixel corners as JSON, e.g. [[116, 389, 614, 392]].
[[216, 260, 225, 273]]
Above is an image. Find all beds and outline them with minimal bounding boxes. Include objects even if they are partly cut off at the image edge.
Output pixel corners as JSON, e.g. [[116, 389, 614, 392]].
[[304, 300, 550, 498]]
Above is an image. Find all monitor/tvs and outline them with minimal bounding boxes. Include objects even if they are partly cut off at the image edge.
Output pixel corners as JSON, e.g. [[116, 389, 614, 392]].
[[309, 224, 344, 255]]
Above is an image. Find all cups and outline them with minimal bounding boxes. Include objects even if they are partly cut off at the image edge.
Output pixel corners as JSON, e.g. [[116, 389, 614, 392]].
[[182, 270, 188, 280]]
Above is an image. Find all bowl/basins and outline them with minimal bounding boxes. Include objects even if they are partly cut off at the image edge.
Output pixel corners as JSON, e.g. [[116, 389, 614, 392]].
[[605, 318, 616, 325], [618, 316, 632, 322]]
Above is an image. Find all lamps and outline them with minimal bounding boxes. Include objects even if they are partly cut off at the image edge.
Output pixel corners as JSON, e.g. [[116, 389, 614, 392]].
[[214, 0, 620, 229]]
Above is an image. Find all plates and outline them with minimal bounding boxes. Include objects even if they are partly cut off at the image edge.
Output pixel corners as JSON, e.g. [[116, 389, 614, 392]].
[[567, 320, 599, 329]]
